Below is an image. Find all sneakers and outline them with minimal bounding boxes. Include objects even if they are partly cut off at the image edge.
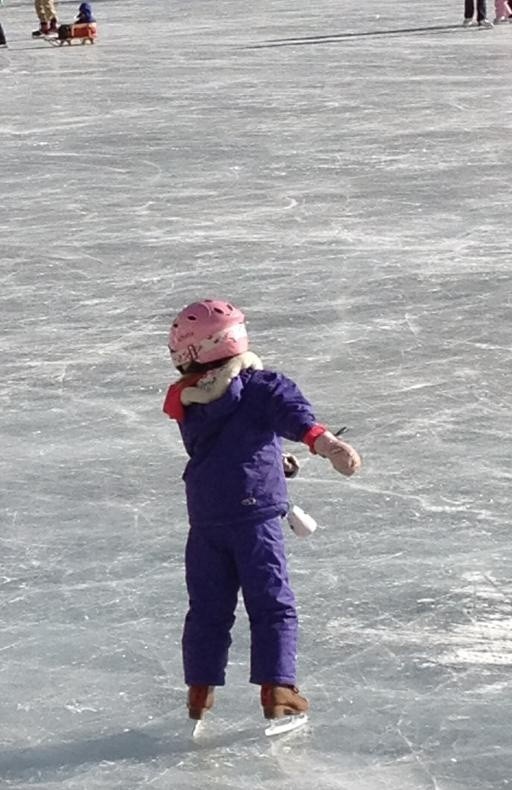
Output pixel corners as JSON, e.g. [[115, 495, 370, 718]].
[[186, 686, 214, 719], [463, 16, 511, 30], [261, 684, 309, 718]]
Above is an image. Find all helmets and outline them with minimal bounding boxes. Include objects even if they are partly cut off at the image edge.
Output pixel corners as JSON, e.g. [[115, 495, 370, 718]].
[[168, 300, 248, 372]]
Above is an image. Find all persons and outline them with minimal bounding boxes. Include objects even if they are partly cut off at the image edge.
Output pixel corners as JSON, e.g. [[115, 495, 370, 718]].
[[32, 0, 59, 36], [162, 299, 362, 720], [74, 3, 96, 24], [462, 0, 512, 27]]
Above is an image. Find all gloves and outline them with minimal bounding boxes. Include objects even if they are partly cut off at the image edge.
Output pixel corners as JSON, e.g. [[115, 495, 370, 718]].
[[314, 430, 360, 477], [281, 452, 300, 479]]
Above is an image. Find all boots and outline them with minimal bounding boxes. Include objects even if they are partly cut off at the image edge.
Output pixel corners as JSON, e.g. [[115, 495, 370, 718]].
[[31, 19, 58, 35]]
